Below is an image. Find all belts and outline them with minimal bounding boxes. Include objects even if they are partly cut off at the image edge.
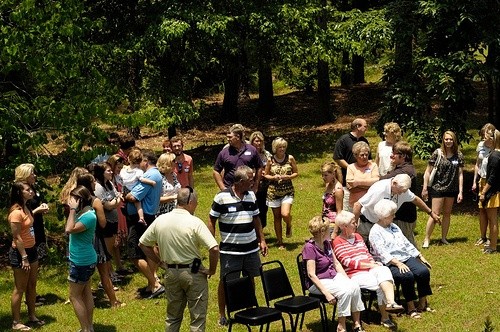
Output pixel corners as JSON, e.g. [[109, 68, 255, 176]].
[[167, 263, 190, 269]]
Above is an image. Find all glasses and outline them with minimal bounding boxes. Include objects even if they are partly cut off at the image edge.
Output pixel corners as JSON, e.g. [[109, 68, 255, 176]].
[[178, 162, 182, 173], [186, 185, 193, 204]]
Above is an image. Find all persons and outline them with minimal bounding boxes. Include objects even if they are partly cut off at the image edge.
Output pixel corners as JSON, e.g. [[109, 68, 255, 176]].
[[380, 141, 417, 246], [302, 216, 366, 332], [353, 175, 442, 246], [422, 131, 464, 247], [375, 123, 401, 175], [65, 186, 97, 332], [321, 161, 344, 241], [138, 185, 219, 332], [58, 133, 194, 308], [333, 118, 372, 210], [346, 142, 379, 212], [472, 123, 500, 253], [368, 199, 434, 318], [208, 166, 268, 325], [7, 163, 47, 331], [213, 124, 299, 249], [332, 211, 405, 327]]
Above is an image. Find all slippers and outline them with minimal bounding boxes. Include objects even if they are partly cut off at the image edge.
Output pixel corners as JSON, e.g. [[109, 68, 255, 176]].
[[417, 305, 436, 313], [407, 310, 421, 319], [14, 326, 32, 332], [29, 319, 45, 325]]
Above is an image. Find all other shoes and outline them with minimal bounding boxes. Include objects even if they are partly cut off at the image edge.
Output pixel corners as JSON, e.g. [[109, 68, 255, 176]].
[[386, 303, 403, 312], [423, 240, 431, 248], [485, 249, 497, 254], [219, 317, 228, 327], [381, 320, 395, 327], [441, 239, 448, 245], [475, 239, 487, 245], [109, 273, 124, 282], [148, 285, 165, 298], [286, 226, 292, 238], [112, 302, 126, 309], [352, 324, 364, 332], [98, 282, 118, 291], [279, 242, 285, 250]]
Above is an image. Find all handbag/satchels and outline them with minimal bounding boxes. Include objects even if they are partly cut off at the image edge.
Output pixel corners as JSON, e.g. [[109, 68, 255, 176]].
[[426, 149, 442, 188]]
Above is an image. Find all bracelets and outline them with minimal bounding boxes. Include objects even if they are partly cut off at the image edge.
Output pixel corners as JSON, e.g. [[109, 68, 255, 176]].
[[428, 211, 432, 215], [22, 255, 28, 260], [71, 207, 76, 210]]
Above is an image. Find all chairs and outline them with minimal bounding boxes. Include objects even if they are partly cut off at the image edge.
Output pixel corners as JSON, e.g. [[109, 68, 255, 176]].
[[222, 252, 403, 332]]
